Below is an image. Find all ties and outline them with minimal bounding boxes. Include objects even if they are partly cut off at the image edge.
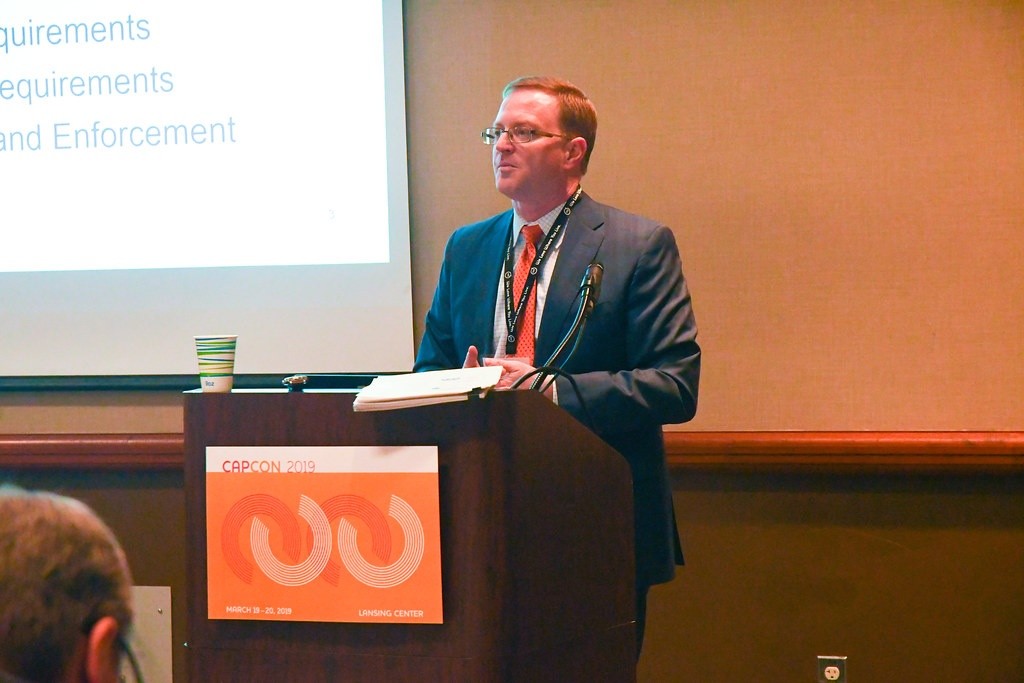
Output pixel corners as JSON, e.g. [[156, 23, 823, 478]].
[[505, 224, 544, 367]]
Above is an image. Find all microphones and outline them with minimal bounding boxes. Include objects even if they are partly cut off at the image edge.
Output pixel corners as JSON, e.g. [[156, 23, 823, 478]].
[[583, 262, 604, 317]]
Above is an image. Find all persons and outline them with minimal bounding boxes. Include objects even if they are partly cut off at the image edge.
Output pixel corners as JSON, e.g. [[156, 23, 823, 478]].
[[413, 77, 701, 683], [0, 486, 147, 682]]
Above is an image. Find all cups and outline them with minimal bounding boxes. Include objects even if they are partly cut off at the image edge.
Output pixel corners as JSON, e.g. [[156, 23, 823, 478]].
[[194, 335, 238, 393]]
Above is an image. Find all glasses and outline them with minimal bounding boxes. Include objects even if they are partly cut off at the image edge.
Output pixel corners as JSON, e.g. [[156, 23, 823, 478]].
[[481, 128, 572, 145]]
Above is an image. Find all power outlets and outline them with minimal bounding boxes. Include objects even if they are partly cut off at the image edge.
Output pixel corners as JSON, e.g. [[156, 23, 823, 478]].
[[817, 656, 848, 683]]
